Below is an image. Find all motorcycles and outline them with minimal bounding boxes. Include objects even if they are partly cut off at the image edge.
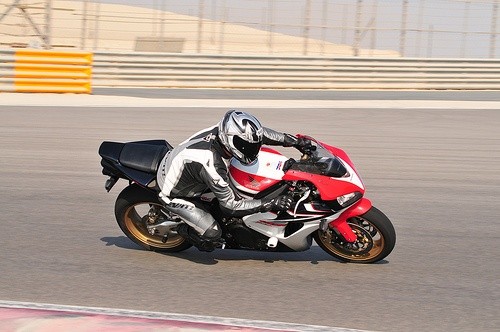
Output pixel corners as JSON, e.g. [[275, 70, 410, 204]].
[[98, 132, 396, 263]]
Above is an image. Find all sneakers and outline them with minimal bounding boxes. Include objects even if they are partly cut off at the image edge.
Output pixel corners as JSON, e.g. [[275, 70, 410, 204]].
[[177, 224, 218, 253]]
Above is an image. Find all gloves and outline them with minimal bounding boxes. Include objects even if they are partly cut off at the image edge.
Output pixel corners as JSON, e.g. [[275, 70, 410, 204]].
[[261, 194, 295, 213], [283, 133, 317, 156]]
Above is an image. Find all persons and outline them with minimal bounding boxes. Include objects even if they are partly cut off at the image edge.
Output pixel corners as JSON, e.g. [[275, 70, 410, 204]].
[[155, 107, 312, 255]]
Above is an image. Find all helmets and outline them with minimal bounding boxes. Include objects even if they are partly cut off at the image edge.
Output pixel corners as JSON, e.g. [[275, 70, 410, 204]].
[[217, 110, 264, 164]]
